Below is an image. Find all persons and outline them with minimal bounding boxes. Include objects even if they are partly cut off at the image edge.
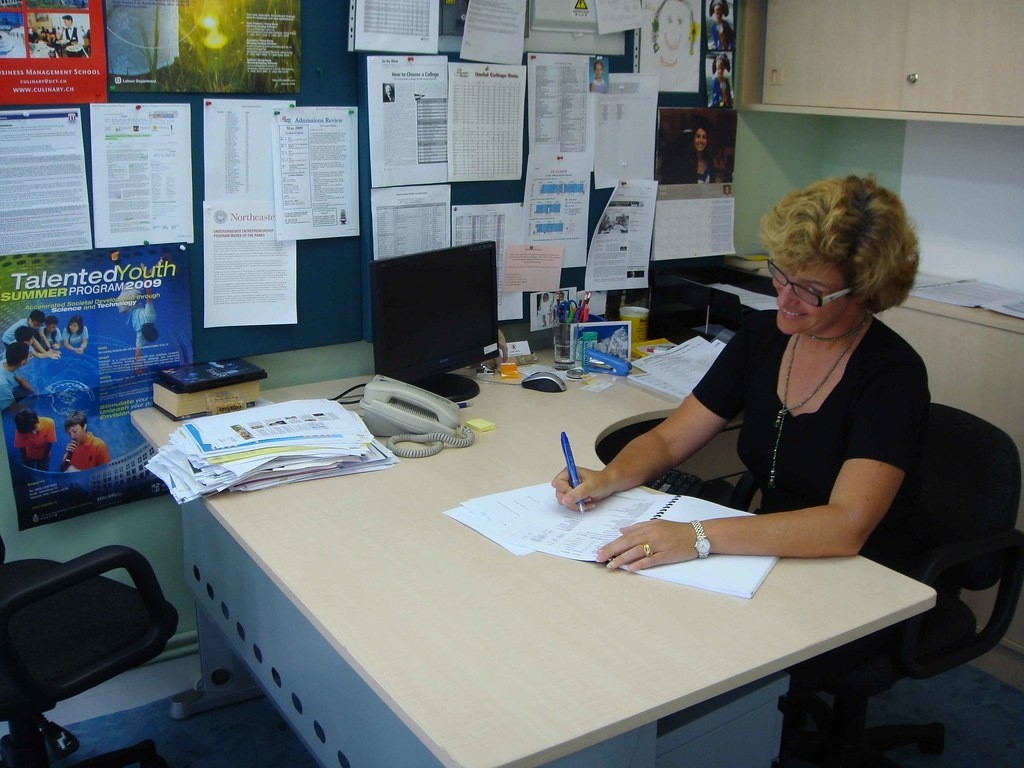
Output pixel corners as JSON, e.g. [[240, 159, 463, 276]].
[[553, 175, 933, 768], [709, 0, 733, 50], [538, 291, 564, 327], [674, 124, 718, 183], [710, 54, 732, 107], [383, 84, 395, 102], [28, 15, 91, 58], [590, 60, 606, 93], [61, 412, 110, 472], [0, 309, 88, 413], [111, 251, 168, 375], [15, 409, 57, 471]]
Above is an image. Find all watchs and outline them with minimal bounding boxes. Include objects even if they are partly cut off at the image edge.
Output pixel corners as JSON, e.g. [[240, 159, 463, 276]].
[[691, 520, 711, 559]]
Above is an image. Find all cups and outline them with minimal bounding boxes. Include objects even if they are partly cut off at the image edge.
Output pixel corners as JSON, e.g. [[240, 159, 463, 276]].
[[552, 321, 572, 370], [620, 306, 649, 342]]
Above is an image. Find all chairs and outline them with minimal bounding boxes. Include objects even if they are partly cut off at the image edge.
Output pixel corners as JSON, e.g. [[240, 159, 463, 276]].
[[781, 403, 1024, 768], [0, 535, 179, 768]]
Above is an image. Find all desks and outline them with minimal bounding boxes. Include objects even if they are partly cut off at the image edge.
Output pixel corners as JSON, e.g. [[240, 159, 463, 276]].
[[131, 340, 938, 768]]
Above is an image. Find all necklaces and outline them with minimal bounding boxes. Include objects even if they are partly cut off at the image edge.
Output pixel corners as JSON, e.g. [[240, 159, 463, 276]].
[[769, 314, 871, 490]]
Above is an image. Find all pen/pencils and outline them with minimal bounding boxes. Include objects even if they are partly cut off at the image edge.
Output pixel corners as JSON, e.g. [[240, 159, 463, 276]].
[[646, 348, 668, 353], [560, 431, 587, 516], [456, 402, 474, 409], [566, 290, 593, 338]]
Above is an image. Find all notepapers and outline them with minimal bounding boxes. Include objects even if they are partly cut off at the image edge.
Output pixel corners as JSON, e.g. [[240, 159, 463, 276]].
[[465, 418, 497, 433]]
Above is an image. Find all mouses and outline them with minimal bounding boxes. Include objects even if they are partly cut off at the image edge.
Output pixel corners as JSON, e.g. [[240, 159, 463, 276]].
[[522, 372, 567, 393]]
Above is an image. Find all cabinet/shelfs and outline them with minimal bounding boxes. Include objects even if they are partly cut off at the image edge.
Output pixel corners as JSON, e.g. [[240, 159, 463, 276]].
[[755, 266, 1024, 688], [739, 0, 1024, 126]]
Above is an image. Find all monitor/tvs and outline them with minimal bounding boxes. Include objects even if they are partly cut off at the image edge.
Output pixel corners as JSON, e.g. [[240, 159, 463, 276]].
[[369, 240, 500, 403]]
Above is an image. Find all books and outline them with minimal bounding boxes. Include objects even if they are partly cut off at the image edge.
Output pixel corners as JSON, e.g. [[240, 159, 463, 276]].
[[151, 379, 260, 422], [506, 486, 781, 598], [627, 373, 689, 401], [632, 338, 678, 358]]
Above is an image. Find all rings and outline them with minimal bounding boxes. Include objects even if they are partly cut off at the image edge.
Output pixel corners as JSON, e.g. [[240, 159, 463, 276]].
[[641, 543, 654, 559]]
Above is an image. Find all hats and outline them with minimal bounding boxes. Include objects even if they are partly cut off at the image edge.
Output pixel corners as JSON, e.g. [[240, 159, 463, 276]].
[[711, 52, 731, 75], [709, 0, 730, 18]]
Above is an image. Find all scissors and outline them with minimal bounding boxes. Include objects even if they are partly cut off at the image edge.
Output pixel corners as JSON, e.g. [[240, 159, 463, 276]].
[[556, 299, 578, 340]]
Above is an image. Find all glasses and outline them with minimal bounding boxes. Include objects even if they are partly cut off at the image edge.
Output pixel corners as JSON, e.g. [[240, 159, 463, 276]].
[[767, 258, 854, 308]]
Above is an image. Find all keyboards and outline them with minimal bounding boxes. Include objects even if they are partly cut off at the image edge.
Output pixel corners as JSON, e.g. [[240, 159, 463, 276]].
[[644, 469, 705, 498]]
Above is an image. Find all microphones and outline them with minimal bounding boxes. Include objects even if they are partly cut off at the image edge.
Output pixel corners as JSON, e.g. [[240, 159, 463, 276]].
[[65, 442, 77, 463]]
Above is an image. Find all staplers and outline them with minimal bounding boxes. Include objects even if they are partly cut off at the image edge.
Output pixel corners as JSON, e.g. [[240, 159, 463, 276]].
[[583, 347, 633, 378]]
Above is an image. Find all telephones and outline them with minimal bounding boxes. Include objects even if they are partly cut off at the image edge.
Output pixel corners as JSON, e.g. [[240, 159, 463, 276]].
[[358, 374, 461, 438]]
